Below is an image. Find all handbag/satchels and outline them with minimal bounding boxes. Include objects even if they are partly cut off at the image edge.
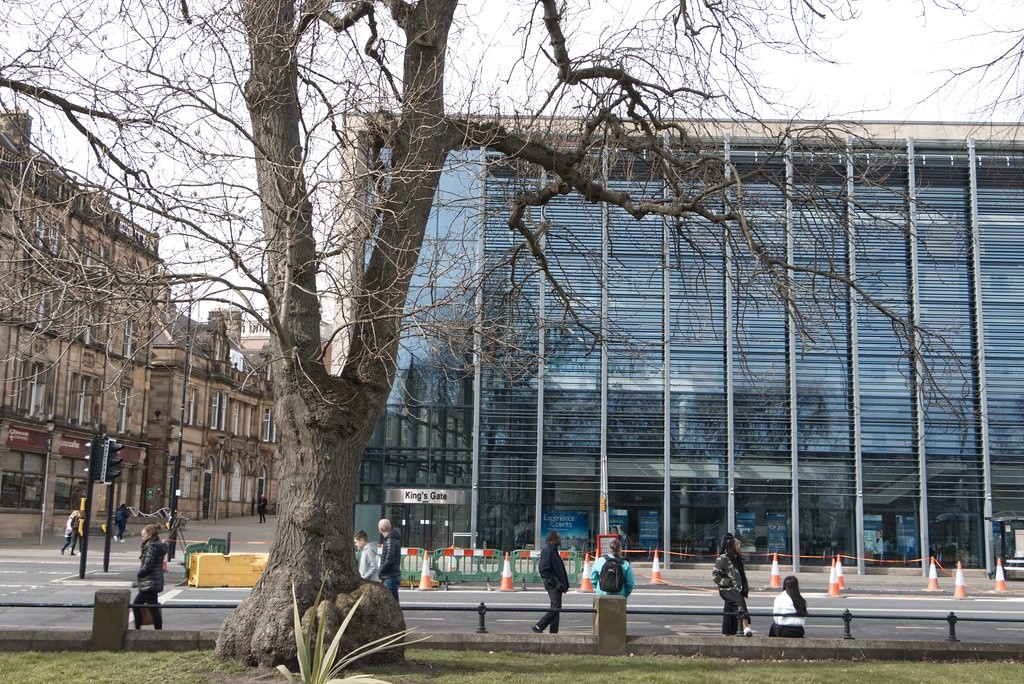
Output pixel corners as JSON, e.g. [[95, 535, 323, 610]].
[[121, 509, 130, 518], [140, 603, 162, 625]]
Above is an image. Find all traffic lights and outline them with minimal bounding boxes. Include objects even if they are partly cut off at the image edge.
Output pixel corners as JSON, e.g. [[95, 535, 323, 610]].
[[101, 438, 124, 485], [84, 438, 104, 481]]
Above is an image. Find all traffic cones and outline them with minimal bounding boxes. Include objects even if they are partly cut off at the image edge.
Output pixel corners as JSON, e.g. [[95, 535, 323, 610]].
[[824, 559, 847, 598], [418, 550, 437, 591], [995, 558, 1009, 593], [495, 551, 518, 592], [921, 556, 946, 592], [765, 553, 782, 587], [836, 555, 851, 589], [647, 550, 668, 585], [576, 553, 597, 592], [944, 561, 975, 600]]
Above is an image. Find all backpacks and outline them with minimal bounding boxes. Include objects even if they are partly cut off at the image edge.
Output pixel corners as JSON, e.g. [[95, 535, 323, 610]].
[[598, 555, 630, 592]]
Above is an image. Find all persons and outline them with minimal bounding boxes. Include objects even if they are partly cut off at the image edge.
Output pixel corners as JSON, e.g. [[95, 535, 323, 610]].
[[354, 530, 379, 583], [531, 531, 570, 634], [113, 504, 129, 543], [258, 494, 268, 523], [378, 518, 402, 603], [768, 576, 808, 638], [870, 530, 882, 567], [590, 540, 635, 600], [611, 525, 622, 540], [61, 510, 84, 556], [621, 531, 632, 556], [712, 538, 754, 637], [132, 522, 169, 630]]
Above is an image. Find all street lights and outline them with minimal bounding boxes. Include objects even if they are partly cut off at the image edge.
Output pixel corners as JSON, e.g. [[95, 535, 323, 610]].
[[151, 360, 188, 562]]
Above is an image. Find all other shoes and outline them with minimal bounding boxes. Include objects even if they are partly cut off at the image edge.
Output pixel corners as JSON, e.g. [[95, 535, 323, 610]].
[[78, 552, 81, 556], [121, 539, 126, 543], [114, 535, 118, 543], [61, 548, 64, 555], [531, 626, 544, 633], [743, 627, 753, 637], [258, 522, 262, 523], [71, 551, 76, 555]]
